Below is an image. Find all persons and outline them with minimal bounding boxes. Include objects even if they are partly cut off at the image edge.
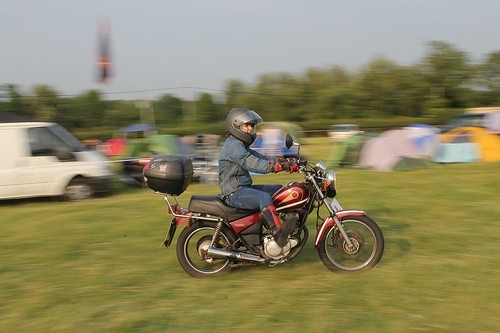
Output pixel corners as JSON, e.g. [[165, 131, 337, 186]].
[[218, 107, 298, 248]]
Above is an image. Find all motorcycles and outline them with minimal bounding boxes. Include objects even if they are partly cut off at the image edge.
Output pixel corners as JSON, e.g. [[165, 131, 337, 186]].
[[143, 133, 385, 280]]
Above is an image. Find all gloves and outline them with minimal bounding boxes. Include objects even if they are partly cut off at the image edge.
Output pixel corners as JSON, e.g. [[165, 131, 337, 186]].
[[275, 160, 299, 173]]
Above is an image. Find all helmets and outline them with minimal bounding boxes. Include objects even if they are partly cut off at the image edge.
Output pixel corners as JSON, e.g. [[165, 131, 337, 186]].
[[225, 108, 263, 145]]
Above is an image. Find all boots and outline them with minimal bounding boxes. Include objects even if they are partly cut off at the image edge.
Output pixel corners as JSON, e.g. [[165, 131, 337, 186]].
[[261, 209, 297, 247]]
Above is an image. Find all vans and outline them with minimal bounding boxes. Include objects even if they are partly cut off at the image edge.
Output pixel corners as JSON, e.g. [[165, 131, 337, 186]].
[[0, 122, 112, 201]]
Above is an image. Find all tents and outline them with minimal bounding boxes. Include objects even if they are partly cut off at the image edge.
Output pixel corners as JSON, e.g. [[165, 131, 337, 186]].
[[330, 109, 500, 170]]
